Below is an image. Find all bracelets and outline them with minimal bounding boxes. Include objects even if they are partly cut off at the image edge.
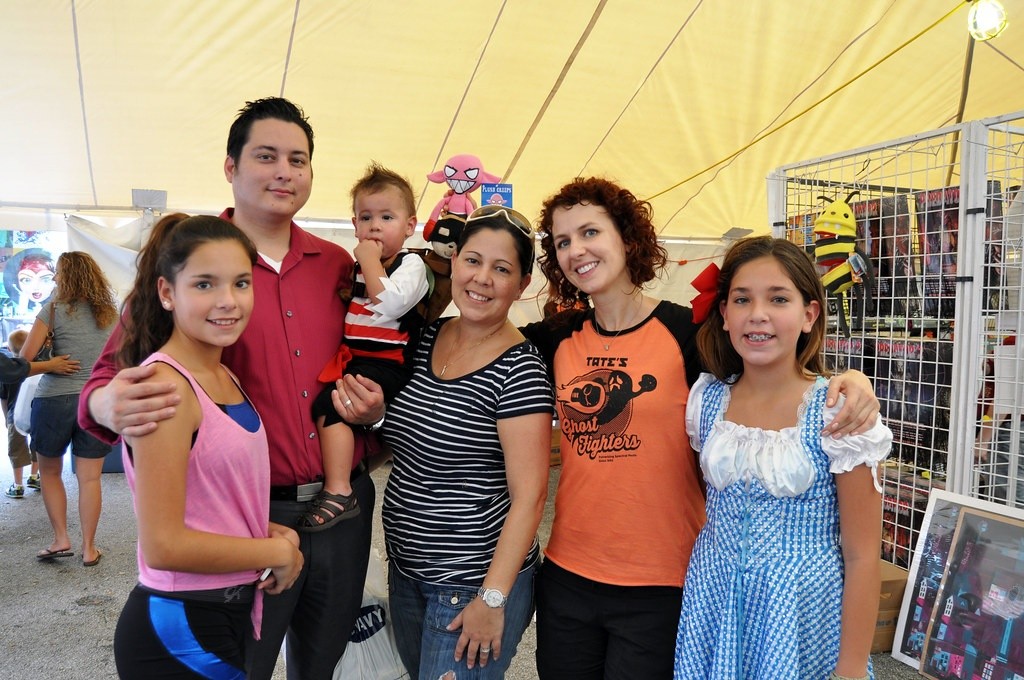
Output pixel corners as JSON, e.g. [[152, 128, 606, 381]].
[[829, 671, 870, 680]]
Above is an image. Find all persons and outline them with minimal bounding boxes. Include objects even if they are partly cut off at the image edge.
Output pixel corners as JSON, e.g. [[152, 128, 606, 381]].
[[295, 167, 430, 532], [0, 329, 40, 498], [380, 205, 555, 680], [0, 353, 81, 383], [19, 251, 120, 566], [114, 212, 303, 680], [676, 237, 894, 680], [78, 98, 385, 680], [518, 177, 881, 680]]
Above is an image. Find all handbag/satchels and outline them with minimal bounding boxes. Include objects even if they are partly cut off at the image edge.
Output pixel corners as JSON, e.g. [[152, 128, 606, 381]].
[[31, 300, 56, 374], [331, 546, 411, 680], [12, 374, 46, 437]]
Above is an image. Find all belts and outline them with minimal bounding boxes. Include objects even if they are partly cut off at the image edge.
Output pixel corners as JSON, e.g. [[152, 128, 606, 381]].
[[270, 461, 365, 502]]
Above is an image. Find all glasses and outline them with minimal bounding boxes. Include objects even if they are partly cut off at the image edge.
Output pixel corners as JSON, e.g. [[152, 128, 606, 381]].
[[463, 205, 535, 273]]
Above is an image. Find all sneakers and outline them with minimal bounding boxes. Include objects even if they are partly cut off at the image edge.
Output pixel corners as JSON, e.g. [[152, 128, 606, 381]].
[[26, 474, 40, 490], [5, 483, 24, 497]]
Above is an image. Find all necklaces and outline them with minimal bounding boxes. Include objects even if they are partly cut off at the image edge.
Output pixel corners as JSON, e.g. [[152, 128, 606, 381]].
[[440, 335, 491, 376], [595, 292, 643, 351]]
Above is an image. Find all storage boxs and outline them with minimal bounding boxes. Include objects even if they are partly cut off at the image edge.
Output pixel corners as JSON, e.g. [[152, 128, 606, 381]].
[[878, 559, 909, 611], [870, 609, 900, 654]]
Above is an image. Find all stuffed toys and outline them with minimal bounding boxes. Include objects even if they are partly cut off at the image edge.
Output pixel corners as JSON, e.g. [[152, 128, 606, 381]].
[[426, 214, 466, 261], [421, 155, 502, 242]]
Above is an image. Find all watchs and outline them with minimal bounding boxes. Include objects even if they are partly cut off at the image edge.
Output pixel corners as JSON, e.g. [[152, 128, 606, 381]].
[[477, 587, 507, 608], [362, 416, 384, 432]]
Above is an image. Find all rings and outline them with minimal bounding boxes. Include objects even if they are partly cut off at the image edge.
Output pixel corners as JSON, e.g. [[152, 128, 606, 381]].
[[481, 647, 490, 652], [344, 400, 351, 408]]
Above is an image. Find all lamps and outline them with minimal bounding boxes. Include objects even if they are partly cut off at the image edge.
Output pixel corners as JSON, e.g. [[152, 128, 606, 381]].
[[968, 0, 1010, 42]]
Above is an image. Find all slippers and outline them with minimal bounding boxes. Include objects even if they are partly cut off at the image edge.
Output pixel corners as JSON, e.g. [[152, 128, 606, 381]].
[[81, 549, 102, 566], [36, 546, 74, 559]]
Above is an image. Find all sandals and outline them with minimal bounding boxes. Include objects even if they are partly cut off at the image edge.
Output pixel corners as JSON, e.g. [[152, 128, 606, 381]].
[[297, 491, 361, 532]]
